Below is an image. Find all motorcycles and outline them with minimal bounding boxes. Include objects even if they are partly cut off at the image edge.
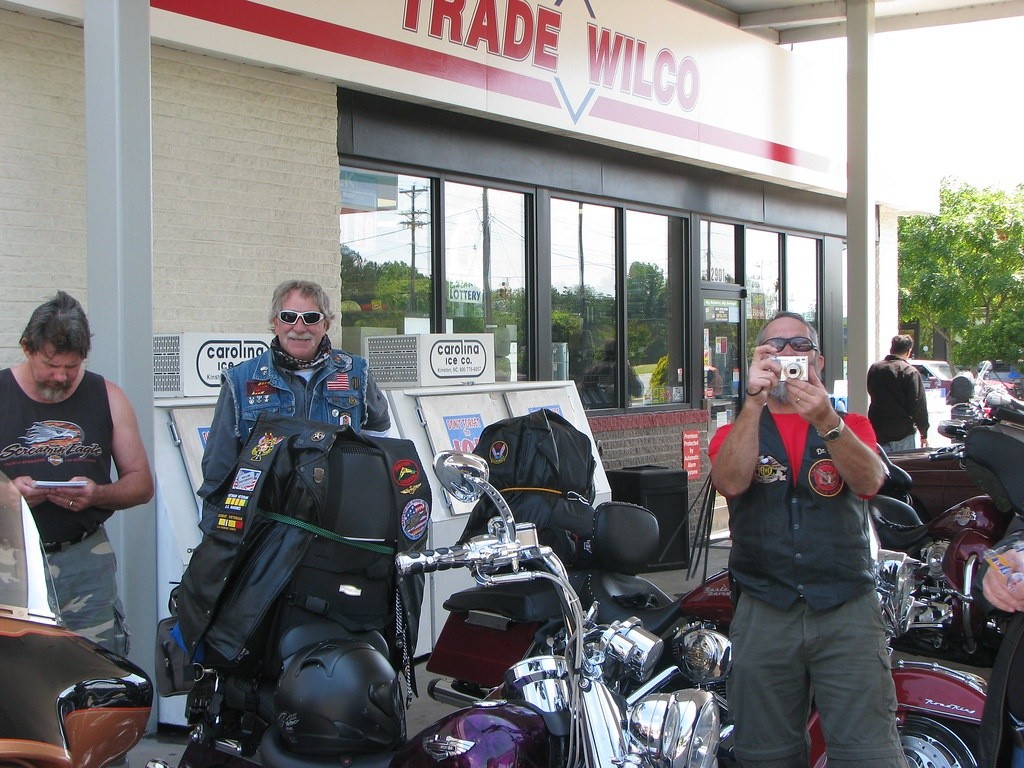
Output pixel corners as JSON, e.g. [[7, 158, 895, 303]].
[[1, 394, 1024, 768]]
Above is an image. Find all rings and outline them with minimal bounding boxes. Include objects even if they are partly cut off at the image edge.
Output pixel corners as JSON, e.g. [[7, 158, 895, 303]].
[[67, 500, 74, 509], [796, 398, 801, 403]]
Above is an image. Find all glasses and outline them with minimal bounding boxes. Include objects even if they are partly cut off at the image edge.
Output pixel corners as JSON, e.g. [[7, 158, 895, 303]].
[[276, 310, 325, 325], [761, 337, 819, 355]]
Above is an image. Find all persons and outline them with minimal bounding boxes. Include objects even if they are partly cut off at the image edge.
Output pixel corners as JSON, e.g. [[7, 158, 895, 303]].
[[974, 513, 1024, 768], [867, 334, 930, 452], [0, 290, 153, 768], [708, 312, 909, 768], [178, 279, 392, 768], [707, 366, 724, 398], [576, 338, 645, 409]]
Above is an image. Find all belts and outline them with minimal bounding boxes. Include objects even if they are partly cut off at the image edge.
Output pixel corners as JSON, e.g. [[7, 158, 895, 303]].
[[44, 524, 100, 554]]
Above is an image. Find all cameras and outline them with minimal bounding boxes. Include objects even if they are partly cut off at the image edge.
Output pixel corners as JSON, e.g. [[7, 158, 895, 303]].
[[767, 354, 809, 383]]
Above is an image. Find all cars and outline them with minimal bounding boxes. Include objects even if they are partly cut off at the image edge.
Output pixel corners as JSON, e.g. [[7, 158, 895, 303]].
[[906, 356, 963, 388]]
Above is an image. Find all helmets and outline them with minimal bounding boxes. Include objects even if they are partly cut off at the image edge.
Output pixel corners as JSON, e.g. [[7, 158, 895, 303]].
[[277, 639, 406, 755]]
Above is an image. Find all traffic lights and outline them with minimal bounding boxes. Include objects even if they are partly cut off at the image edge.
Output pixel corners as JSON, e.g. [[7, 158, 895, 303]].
[[498, 287, 506, 299], [506, 287, 513, 299]]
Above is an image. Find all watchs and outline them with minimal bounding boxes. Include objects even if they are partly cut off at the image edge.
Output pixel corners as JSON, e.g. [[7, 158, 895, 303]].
[[817, 417, 844, 442]]
